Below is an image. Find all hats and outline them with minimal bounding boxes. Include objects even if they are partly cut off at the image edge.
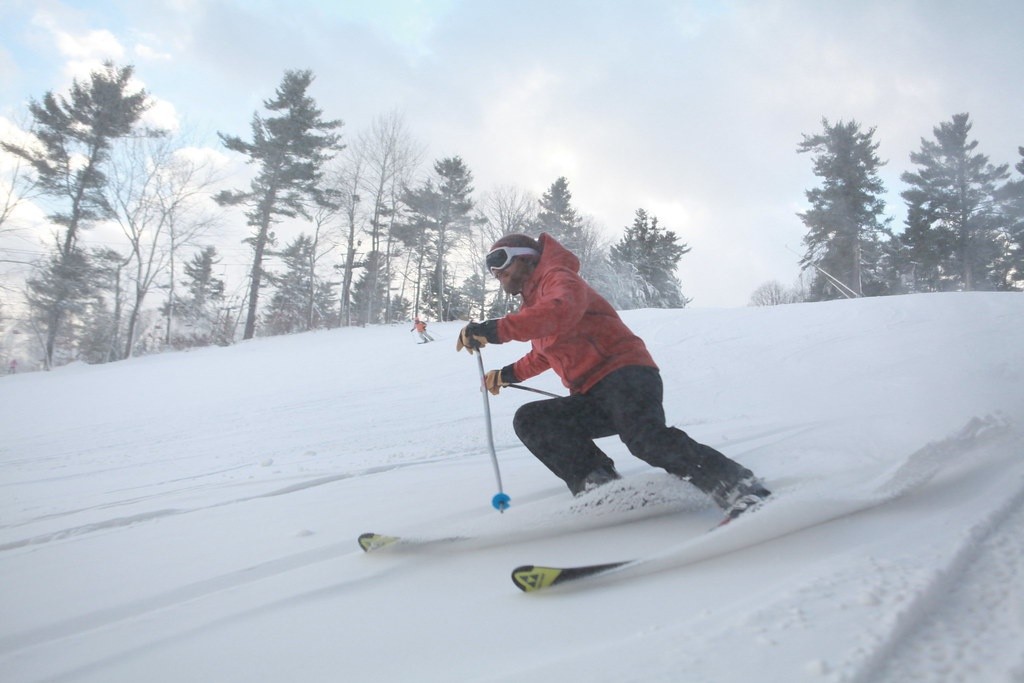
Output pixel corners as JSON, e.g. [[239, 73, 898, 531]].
[[490, 234, 542, 263]]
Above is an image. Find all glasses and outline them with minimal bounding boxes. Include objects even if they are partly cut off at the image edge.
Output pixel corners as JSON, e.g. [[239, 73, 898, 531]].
[[485, 246, 541, 276]]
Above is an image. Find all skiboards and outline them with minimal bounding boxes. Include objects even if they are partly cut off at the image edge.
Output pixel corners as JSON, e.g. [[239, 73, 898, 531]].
[[357, 503, 740, 594]]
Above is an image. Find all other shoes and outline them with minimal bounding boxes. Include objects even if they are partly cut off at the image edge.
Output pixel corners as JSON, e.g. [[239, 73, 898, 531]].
[[716, 469, 772, 527]]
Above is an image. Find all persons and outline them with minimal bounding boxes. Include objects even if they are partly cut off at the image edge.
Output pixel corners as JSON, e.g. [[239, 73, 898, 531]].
[[456, 232, 773, 518], [410, 317, 434, 343]]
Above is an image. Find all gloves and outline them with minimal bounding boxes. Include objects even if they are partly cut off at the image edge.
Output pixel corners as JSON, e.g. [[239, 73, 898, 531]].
[[480, 364, 522, 395], [456, 319, 503, 356]]
